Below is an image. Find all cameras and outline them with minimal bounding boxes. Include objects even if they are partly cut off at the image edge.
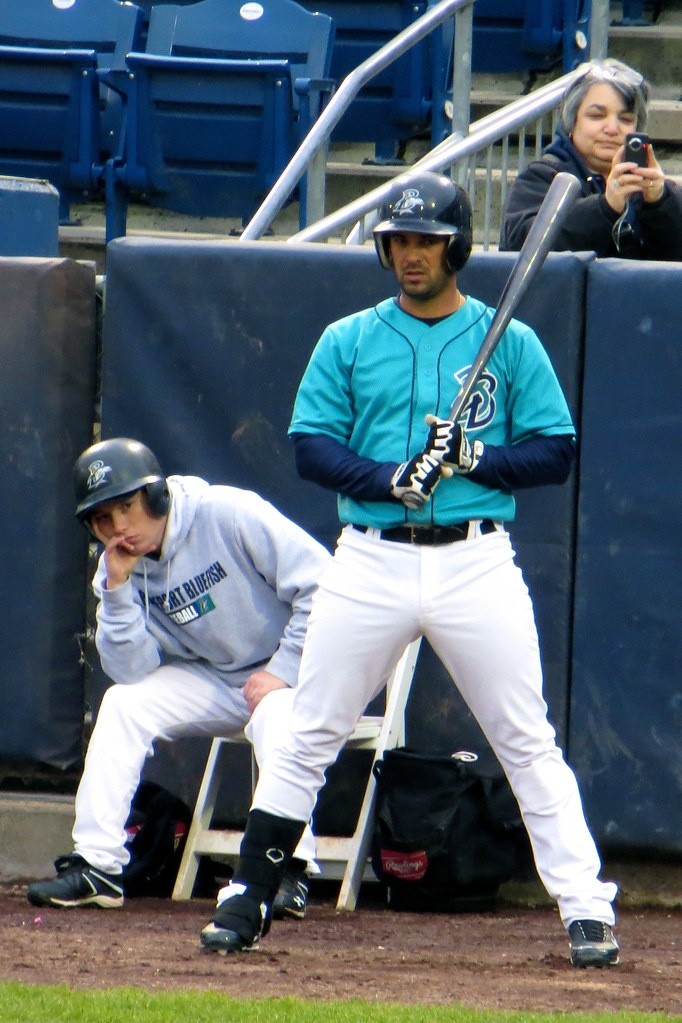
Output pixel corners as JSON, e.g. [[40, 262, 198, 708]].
[[625, 133, 649, 174]]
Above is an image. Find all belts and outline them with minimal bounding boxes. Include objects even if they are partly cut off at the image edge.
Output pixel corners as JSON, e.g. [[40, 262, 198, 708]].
[[350, 518, 498, 546]]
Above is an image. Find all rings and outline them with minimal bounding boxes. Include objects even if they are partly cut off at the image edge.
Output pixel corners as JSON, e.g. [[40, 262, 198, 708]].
[[650, 180, 652, 187]]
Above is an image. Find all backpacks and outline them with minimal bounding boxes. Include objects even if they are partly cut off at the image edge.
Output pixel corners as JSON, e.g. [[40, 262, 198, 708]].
[[123, 778, 235, 899], [368, 745, 526, 917]]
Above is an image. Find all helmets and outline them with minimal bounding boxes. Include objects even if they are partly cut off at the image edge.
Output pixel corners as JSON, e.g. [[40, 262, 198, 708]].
[[72, 438, 170, 535], [371, 172, 474, 272]]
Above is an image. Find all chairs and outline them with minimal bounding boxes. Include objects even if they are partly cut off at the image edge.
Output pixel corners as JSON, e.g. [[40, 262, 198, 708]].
[[0, 0, 647, 262], [171, 633, 423, 913]]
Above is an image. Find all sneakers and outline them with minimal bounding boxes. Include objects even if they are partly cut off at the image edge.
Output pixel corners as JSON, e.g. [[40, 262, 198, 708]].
[[271, 856, 315, 923], [27, 852, 125, 908], [566, 920, 619, 968], [199, 875, 274, 955]]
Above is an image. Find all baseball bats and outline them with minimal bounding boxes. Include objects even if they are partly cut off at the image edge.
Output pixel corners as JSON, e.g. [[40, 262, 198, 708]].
[[402, 171, 585, 510]]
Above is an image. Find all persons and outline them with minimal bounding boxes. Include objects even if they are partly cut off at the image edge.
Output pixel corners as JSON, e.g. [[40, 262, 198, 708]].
[[27, 437, 333, 921], [498, 59, 682, 262], [199, 171, 620, 969]]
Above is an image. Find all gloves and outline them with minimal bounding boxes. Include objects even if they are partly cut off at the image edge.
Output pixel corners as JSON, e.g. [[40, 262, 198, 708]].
[[390, 451, 453, 504], [424, 411, 485, 474]]
